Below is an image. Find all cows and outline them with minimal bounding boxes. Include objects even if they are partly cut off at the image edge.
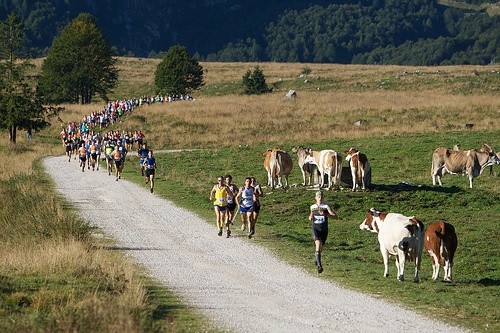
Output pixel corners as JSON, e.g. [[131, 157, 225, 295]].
[[359, 208, 424, 283], [344, 148, 370, 191], [424, 222, 457, 282], [263, 148, 293, 190], [431, 144, 500, 188], [292, 145, 342, 190]]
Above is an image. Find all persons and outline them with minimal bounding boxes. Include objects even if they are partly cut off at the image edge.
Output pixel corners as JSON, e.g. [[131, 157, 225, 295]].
[[59, 94, 196, 193], [210, 175, 263, 239], [308, 191, 336, 273]]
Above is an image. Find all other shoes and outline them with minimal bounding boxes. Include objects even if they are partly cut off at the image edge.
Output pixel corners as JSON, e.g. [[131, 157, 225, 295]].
[[248, 231, 253, 237], [315, 261, 317, 266], [241, 225, 246, 231], [218, 229, 222, 236], [226, 231, 229, 238], [318, 266, 323, 273], [252, 228, 255, 234]]
